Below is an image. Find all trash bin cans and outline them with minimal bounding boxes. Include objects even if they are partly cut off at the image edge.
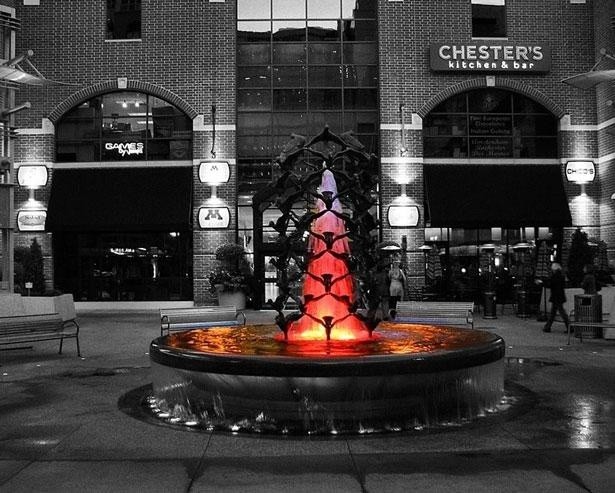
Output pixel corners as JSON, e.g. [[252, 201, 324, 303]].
[[574, 294, 603, 339], [482, 292, 497, 319]]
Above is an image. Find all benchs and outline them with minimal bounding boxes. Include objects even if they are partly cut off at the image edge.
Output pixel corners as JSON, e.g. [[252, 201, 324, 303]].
[[393, 301, 475, 331], [568, 319, 615, 344], [157, 305, 247, 338], [2, 312, 82, 359]]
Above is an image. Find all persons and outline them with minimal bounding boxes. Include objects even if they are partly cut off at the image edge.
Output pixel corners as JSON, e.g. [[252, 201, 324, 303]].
[[581, 262, 598, 294], [387, 260, 407, 318], [535, 263, 576, 333]]
